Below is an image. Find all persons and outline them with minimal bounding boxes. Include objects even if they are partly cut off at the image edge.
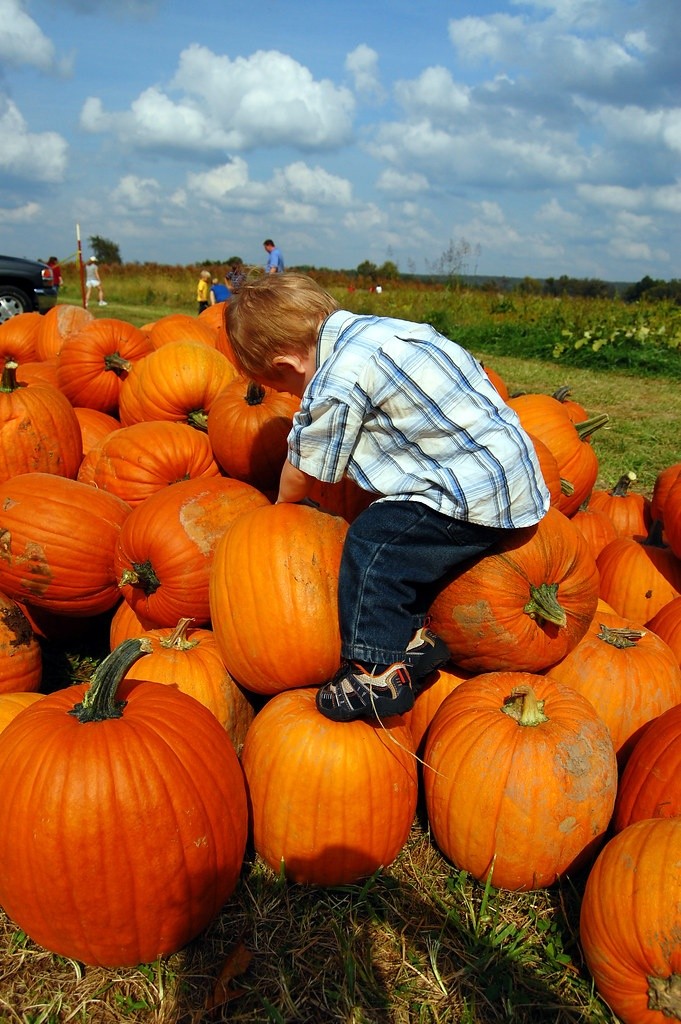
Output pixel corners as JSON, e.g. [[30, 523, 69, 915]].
[[45, 256, 63, 293], [85, 256, 108, 308], [263, 239, 284, 276], [223, 273, 550, 722], [197, 257, 248, 315]]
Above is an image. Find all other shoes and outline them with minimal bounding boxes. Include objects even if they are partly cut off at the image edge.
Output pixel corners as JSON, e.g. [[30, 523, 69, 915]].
[[99, 301, 108, 306]]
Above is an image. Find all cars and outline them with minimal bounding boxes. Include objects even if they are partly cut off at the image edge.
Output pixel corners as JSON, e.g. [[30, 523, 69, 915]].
[[0, 255, 57, 324]]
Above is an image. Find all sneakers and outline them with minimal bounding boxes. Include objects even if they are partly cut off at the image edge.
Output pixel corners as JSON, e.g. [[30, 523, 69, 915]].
[[316, 615, 450, 721]]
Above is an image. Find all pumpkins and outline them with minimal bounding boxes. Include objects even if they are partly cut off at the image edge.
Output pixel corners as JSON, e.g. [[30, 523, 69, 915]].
[[0, 301, 681, 1024]]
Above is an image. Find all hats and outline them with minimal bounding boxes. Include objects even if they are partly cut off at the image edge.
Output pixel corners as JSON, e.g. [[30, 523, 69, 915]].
[[90, 255, 100, 262]]
